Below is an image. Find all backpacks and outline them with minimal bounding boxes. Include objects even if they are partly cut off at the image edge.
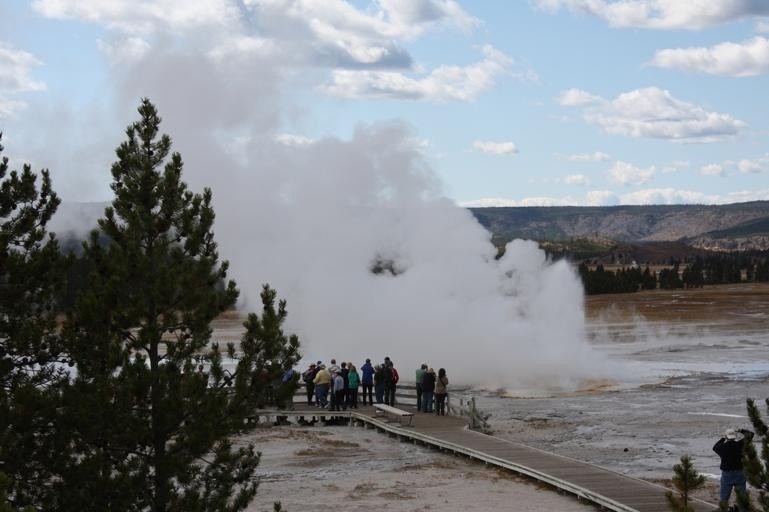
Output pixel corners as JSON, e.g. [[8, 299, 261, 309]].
[[302, 369, 311, 382]]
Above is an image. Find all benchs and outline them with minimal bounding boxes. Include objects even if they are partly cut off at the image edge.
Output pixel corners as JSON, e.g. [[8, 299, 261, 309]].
[[371, 403, 415, 428]]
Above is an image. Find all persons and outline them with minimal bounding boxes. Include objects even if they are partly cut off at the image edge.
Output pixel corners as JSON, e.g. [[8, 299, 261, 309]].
[[712, 427, 755, 505], [302, 356, 400, 411], [422, 367, 436, 412], [433, 368, 449, 415], [416, 363, 428, 411]]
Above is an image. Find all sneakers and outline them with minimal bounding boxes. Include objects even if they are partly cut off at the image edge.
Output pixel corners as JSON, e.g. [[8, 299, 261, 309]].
[[306, 402, 393, 412]]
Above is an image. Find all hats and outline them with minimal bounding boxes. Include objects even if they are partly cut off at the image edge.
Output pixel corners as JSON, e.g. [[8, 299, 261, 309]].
[[725, 429, 737, 439], [308, 362, 340, 374]]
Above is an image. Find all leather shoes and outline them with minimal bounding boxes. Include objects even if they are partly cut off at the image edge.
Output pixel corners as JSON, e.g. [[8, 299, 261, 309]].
[[416, 409, 445, 416]]
